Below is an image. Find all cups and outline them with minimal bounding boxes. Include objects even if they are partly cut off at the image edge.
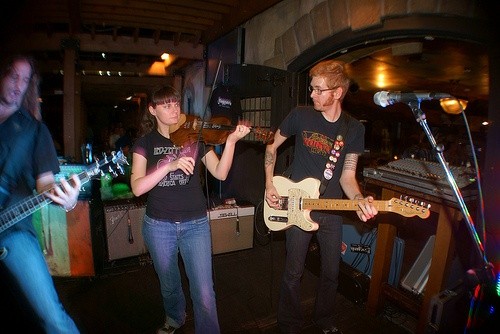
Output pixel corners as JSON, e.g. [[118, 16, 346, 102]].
[[81, 143, 92, 164]]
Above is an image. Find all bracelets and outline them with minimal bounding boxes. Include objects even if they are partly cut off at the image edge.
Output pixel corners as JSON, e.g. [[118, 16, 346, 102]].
[[60, 203, 77, 212]]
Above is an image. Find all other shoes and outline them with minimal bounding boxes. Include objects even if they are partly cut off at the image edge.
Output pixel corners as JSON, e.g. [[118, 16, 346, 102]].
[[158, 317, 186, 334], [320, 326, 340, 334]]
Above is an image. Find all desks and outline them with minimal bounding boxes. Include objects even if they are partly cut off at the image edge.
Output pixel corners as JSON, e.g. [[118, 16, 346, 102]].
[[363, 178, 478, 334]]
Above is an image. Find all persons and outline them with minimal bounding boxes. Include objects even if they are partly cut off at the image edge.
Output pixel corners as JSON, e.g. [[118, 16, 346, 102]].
[[265, 60, 378, 334], [0, 53, 81, 334], [108, 120, 126, 150], [131, 81, 251, 334]]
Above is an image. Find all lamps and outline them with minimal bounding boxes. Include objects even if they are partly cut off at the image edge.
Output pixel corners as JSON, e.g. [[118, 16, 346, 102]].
[[439, 98, 469, 116]]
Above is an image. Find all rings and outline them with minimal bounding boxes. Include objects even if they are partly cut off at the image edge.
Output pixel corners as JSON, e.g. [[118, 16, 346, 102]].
[[239, 128, 243, 132]]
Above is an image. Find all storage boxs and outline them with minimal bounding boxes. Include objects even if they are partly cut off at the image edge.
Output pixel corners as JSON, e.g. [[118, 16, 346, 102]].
[[206, 204, 255, 255]]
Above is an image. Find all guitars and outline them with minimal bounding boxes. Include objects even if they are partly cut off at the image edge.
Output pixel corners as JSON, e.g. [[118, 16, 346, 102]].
[[262, 175, 432, 232], [0, 147, 130, 261]]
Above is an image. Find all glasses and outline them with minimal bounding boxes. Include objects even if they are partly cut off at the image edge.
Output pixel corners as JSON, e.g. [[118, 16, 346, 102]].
[[308, 85, 338, 95]]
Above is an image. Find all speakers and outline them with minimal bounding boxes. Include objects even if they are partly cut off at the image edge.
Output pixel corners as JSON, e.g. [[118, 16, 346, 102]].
[[207, 206, 255, 255], [102, 197, 149, 262], [340, 219, 404, 287], [30, 195, 98, 279]]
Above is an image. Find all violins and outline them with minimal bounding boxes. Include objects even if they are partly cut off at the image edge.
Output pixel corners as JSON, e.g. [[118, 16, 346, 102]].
[[168, 112, 276, 151]]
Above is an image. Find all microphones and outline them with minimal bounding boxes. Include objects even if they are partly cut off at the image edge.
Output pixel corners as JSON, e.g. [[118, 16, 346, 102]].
[[128, 218, 134, 244], [374, 91, 453, 108], [41, 230, 49, 256], [235, 216, 240, 236]]
